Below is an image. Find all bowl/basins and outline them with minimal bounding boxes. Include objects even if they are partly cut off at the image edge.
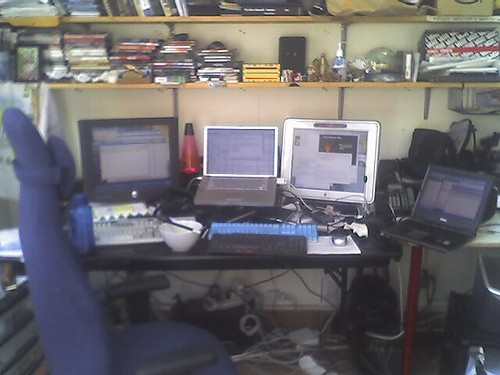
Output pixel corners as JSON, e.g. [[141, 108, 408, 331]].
[[160, 221, 203, 252]]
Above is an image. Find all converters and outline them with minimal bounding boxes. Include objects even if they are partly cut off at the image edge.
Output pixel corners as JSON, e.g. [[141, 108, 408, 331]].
[[289, 327, 319, 347]]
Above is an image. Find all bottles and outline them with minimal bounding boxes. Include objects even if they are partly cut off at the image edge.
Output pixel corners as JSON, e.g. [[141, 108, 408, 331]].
[[69, 194, 95, 257], [180, 123, 199, 173]]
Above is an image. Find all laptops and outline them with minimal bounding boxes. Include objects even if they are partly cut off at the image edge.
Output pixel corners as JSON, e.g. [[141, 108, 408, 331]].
[[194, 124, 278, 207], [382, 163, 496, 253]]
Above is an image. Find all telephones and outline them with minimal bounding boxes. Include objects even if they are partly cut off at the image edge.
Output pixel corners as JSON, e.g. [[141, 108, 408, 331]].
[[376, 178, 417, 212]]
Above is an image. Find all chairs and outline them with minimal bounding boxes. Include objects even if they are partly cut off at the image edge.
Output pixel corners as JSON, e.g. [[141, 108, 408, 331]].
[[1, 109, 237, 375]]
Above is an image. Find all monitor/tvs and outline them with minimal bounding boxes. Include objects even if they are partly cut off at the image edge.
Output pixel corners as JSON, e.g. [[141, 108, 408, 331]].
[[76, 117, 178, 198], [281, 116, 382, 205]]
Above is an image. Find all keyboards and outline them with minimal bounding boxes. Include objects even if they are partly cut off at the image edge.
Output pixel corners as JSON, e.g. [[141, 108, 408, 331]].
[[88, 212, 182, 247], [207, 219, 319, 244], [205, 233, 309, 259]]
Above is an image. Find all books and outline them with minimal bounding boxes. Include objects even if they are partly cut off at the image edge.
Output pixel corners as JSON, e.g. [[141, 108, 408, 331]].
[[63, 32, 110, 79], [218, 2, 243, 15], [188, 1, 219, 15], [242, 63, 280, 82], [152, 40, 197, 82], [109, 38, 158, 83], [419, 56, 499, 83], [0, 0, 188, 16], [196, 49, 241, 83], [18, 32, 69, 78]]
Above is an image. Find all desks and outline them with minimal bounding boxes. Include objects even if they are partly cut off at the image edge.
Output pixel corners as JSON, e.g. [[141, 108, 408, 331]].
[[1, 160, 406, 309], [382, 204, 500, 375]]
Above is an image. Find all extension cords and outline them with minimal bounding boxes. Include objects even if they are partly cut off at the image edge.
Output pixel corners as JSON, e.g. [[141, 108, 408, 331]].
[[260, 298, 297, 313], [200, 280, 256, 311]]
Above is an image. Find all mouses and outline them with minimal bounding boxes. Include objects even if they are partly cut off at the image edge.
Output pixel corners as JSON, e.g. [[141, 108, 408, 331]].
[[332, 227, 349, 247]]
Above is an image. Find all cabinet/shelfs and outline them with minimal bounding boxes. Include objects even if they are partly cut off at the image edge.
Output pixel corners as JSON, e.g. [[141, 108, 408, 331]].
[[0, 13, 500, 122]]
